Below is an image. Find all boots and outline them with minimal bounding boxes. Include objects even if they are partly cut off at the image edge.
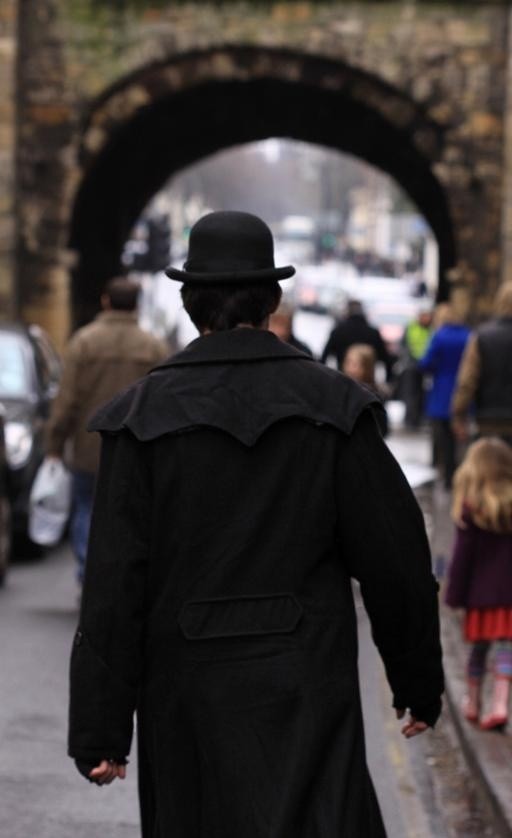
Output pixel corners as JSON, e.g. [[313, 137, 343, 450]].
[[480, 674, 512, 730], [461, 676, 481, 721]]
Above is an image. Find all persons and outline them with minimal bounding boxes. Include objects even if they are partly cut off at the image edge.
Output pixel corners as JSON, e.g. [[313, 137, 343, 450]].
[[45, 276, 170, 592], [403, 288, 470, 492], [320, 298, 387, 384], [267, 290, 314, 356], [447, 278, 512, 462], [440, 434, 512, 732], [62, 203, 448, 835]]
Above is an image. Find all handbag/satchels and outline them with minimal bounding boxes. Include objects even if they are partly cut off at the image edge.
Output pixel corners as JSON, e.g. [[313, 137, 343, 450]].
[[26, 455, 75, 548]]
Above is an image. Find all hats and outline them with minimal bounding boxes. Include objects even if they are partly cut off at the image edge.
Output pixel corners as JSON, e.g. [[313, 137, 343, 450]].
[[164, 209, 296, 284]]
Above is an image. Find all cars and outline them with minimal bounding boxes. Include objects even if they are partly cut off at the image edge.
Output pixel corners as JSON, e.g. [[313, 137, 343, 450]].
[[0, 320, 81, 589]]
